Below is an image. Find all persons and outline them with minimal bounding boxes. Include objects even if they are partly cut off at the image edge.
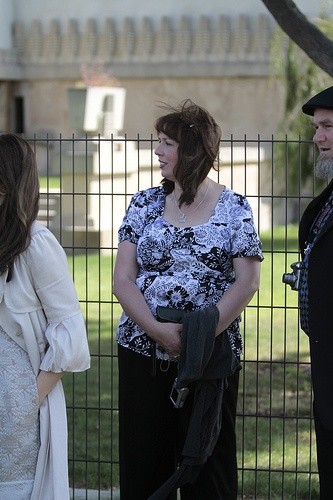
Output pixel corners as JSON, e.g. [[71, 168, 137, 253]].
[[113, 99, 264, 500], [297, 86, 333, 500], [0, 134, 90, 500]]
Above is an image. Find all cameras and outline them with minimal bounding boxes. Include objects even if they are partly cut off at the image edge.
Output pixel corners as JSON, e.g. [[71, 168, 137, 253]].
[[170, 378, 189, 408], [282, 262, 302, 290]]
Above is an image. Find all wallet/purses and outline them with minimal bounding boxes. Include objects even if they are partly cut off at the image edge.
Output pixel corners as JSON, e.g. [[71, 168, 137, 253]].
[[155, 305, 187, 323]]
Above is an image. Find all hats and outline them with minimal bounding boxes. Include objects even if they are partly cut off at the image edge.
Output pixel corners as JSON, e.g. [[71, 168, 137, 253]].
[[303, 87, 333, 115]]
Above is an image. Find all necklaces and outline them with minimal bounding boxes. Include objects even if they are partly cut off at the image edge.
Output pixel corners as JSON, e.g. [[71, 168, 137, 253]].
[[173, 177, 210, 222]]
